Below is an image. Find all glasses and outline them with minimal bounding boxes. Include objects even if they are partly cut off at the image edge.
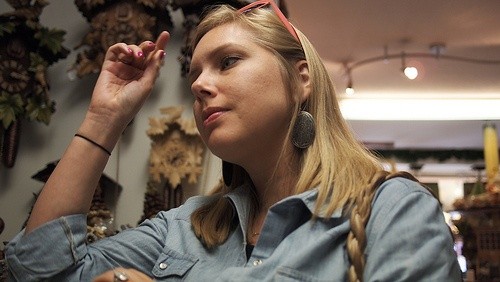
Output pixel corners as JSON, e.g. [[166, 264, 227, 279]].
[[238, 0, 302, 46]]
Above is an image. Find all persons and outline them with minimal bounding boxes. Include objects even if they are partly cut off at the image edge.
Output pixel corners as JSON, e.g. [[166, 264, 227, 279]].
[[3, 1, 465, 282]]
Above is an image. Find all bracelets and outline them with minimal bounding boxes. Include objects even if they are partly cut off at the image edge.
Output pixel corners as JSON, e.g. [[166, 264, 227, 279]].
[[74, 132, 112, 158]]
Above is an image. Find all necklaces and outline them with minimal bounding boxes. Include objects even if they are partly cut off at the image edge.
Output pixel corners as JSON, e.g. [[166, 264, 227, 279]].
[[251, 222, 260, 238]]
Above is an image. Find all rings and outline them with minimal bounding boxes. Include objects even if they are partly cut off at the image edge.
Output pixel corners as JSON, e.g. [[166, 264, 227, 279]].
[[113, 271, 129, 282]]
